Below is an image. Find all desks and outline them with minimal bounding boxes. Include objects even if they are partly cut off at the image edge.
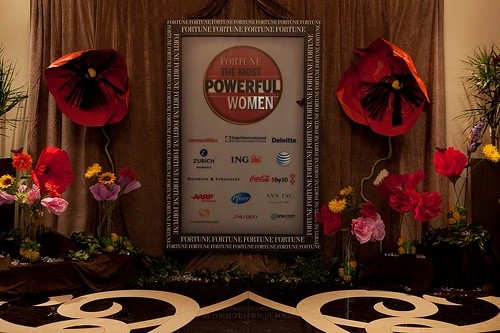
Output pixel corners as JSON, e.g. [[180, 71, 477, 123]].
[[470, 158, 500, 296]]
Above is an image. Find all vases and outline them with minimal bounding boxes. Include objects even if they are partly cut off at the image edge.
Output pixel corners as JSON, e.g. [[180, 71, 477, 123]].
[[0, 253, 138, 295], [378, 245, 436, 289], [338, 228, 361, 282]]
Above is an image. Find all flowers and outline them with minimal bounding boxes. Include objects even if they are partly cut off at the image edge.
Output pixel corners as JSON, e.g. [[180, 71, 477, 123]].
[[137, 255, 340, 290], [451, 41, 500, 158], [0, 144, 143, 265], [316, 147, 500, 289]]
